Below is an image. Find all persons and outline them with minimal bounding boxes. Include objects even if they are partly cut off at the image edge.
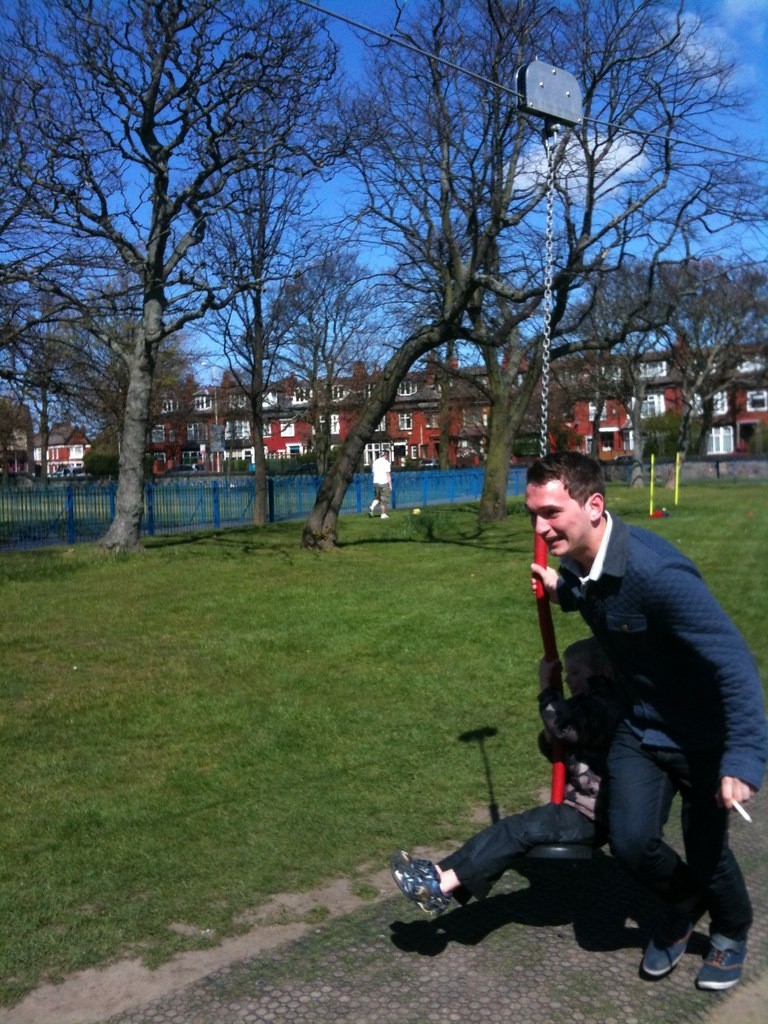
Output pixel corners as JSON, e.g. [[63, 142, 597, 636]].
[[524, 451, 768, 992], [391, 637, 606, 920], [368, 450, 393, 519]]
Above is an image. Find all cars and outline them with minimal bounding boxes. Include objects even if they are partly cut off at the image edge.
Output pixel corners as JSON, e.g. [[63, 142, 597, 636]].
[[418, 457, 438, 471], [163, 464, 206, 478], [49, 465, 83, 481]]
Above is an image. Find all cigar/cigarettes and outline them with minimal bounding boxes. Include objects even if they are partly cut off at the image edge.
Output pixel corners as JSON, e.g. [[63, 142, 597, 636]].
[[731, 799, 753, 824]]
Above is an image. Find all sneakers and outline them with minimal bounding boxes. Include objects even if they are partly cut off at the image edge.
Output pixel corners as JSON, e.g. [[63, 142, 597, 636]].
[[642, 922, 693, 976], [390, 848, 453, 916], [696, 945, 749, 989]]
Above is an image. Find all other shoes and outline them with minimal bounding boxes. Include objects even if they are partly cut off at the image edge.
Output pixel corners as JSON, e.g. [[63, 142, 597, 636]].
[[368, 506, 373, 517], [381, 513, 389, 519]]
